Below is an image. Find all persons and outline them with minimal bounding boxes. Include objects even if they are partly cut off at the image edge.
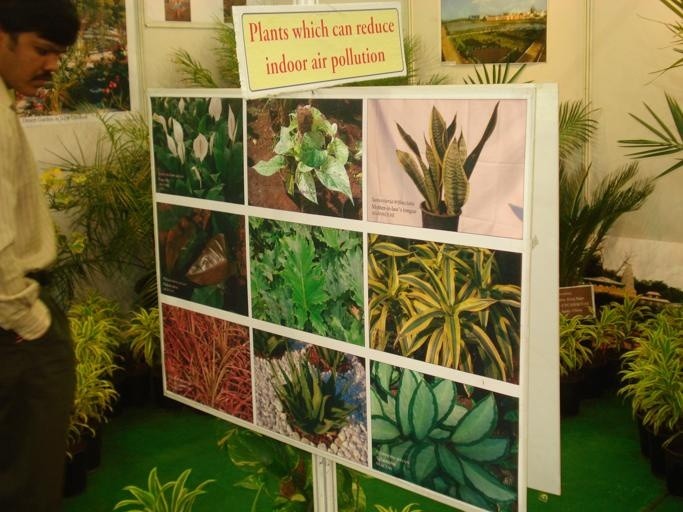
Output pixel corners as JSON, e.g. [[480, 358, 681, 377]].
[[1, 0, 81, 512]]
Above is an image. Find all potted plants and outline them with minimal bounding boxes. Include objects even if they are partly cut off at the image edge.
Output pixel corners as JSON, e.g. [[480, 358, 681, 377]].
[[55, 288, 176, 497], [393, 101, 498, 234], [249, 106, 355, 213], [559, 298, 681, 498], [264, 338, 354, 448]]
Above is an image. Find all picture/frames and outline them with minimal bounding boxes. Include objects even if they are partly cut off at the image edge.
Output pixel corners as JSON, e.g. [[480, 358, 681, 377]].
[[15, 0, 142, 126]]
